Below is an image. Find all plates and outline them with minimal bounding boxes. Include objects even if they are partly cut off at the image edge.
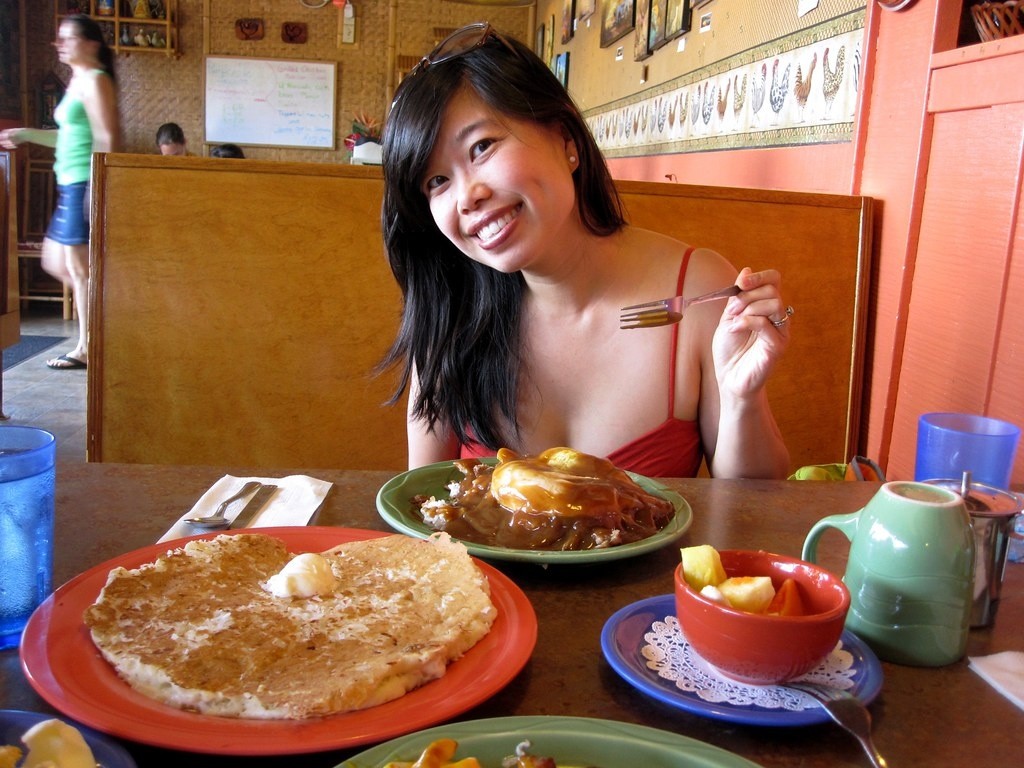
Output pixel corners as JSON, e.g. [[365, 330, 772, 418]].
[[599, 594, 886, 725], [330, 714, 763, 768], [375, 454, 694, 565], [18, 525, 541, 758]]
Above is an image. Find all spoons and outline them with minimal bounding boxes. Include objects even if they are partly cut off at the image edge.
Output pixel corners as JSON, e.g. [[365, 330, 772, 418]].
[[182, 481, 261, 527]]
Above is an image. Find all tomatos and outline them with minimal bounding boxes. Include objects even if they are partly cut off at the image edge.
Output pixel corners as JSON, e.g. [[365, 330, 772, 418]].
[[766, 579, 802, 615]]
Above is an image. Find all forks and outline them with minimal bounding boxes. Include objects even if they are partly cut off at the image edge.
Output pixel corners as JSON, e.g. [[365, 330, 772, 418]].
[[777, 680, 888, 768], [619, 285, 741, 330]]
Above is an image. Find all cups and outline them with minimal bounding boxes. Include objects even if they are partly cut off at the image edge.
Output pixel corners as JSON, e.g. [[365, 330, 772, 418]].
[[0, 425, 57, 652], [800, 480, 979, 668], [916, 478, 1024, 630], [913, 412, 1020, 491]]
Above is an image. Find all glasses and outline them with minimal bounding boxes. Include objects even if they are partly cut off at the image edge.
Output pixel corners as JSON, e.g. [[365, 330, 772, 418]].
[[387, 23, 522, 117]]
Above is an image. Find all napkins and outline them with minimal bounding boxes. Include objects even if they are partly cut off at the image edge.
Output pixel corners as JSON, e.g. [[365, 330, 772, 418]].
[[158, 471, 335, 545], [966, 649, 1024, 712]]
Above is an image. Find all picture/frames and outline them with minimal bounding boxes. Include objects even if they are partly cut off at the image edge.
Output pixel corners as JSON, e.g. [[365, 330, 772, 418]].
[[599, 0, 635, 48], [535, 23, 545, 60]]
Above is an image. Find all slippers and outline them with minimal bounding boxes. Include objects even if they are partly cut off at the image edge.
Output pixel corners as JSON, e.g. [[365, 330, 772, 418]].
[[48, 354, 88, 369]]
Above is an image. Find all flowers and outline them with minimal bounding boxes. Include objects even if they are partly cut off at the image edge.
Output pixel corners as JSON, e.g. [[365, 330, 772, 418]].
[[336, 110, 382, 159]]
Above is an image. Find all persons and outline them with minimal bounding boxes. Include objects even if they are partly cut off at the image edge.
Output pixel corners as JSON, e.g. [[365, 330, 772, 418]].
[[0, 17, 119, 369], [211, 144, 244, 158], [381, 36, 794, 479], [157, 123, 196, 156]]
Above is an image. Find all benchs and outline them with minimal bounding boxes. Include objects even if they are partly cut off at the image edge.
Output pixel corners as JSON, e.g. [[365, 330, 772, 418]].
[[1, 125, 876, 472]]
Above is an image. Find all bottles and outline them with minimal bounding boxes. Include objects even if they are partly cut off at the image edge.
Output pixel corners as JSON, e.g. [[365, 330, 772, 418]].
[[121, 25, 165, 48]]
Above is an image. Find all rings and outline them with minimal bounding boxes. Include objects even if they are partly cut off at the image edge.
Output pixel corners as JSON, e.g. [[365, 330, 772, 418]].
[[772, 305, 793, 327]]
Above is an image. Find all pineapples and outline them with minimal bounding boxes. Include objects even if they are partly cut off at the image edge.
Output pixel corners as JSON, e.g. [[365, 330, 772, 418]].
[[681, 544, 777, 615]]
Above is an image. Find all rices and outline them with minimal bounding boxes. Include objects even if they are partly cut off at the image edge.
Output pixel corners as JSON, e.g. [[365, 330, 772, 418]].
[[422, 482, 488, 529]]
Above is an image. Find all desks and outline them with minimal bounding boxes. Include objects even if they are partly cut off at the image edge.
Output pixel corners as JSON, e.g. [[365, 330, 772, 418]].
[[0, 459, 1024, 768]]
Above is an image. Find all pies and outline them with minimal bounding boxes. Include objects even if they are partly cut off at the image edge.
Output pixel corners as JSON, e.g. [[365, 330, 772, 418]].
[[84, 532, 499, 719]]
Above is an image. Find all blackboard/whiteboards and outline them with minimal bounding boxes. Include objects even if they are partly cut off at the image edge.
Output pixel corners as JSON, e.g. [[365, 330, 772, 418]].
[[203, 54, 338, 150]]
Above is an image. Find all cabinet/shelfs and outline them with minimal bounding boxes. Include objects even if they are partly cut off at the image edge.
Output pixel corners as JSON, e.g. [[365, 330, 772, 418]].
[[54, 0, 179, 61]]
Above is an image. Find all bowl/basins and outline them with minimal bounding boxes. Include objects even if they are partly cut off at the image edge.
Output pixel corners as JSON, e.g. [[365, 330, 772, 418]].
[[673, 547, 852, 689], [0, 709, 134, 768]]
